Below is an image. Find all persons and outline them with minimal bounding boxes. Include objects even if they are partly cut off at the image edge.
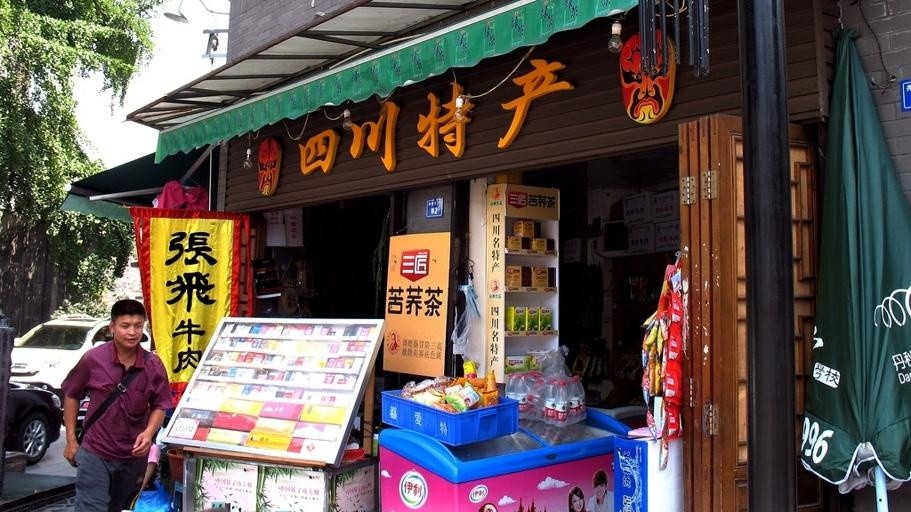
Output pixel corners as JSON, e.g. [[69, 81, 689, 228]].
[[128, 443, 172, 512], [586, 469, 616, 512], [61, 299, 172, 512], [567, 486, 587, 512]]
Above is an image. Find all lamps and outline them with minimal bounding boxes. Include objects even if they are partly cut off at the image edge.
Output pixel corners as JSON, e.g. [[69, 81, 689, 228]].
[[163, 0, 233, 24]]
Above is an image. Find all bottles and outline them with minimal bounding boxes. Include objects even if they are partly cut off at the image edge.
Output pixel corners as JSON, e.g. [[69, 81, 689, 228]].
[[504, 368, 588, 428]]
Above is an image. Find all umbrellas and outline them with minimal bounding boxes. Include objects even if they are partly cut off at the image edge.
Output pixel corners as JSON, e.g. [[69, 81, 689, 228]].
[[799, 26, 911, 512]]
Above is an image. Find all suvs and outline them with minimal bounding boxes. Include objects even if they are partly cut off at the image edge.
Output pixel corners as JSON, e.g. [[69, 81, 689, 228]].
[[3, 316, 150, 377]]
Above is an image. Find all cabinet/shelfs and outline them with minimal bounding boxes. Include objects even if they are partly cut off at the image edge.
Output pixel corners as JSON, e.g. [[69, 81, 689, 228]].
[[489, 184, 561, 385]]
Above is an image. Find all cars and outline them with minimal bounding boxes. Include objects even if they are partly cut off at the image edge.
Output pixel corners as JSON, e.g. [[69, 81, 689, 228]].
[[4, 384, 69, 460], [72, 400, 91, 443]]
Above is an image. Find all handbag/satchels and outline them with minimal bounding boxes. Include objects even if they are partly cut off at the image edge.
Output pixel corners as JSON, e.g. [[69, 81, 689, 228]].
[[68, 428, 83, 467]]
